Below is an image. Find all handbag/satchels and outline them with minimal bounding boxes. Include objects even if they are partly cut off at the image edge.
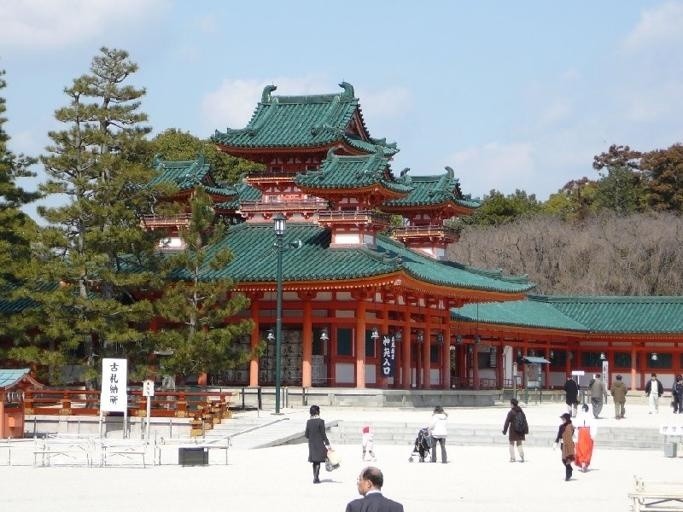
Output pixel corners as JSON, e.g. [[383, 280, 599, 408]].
[[326, 446, 341, 472]]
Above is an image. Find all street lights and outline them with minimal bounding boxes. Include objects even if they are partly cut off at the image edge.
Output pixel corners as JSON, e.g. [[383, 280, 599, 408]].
[[270, 214, 303, 416], [270, 211, 303, 416]]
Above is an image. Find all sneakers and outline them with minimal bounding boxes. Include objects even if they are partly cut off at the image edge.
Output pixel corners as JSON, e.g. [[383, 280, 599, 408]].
[[511, 460, 516, 462], [519, 458, 524, 463]]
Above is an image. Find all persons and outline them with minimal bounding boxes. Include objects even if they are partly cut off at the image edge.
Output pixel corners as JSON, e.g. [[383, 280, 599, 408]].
[[347, 467, 403, 512], [362, 425, 378, 463], [645, 373, 663, 414], [611, 374, 627, 419], [675, 376, 683, 414], [672, 375, 680, 413], [563, 374, 579, 416], [502, 398, 529, 463], [573, 404, 593, 472], [589, 373, 607, 418], [430, 407, 448, 464], [552, 413, 575, 481], [305, 405, 331, 484]]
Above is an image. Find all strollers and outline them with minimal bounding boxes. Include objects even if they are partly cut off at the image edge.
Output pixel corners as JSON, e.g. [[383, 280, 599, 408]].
[[408, 428, 437, 463]]
